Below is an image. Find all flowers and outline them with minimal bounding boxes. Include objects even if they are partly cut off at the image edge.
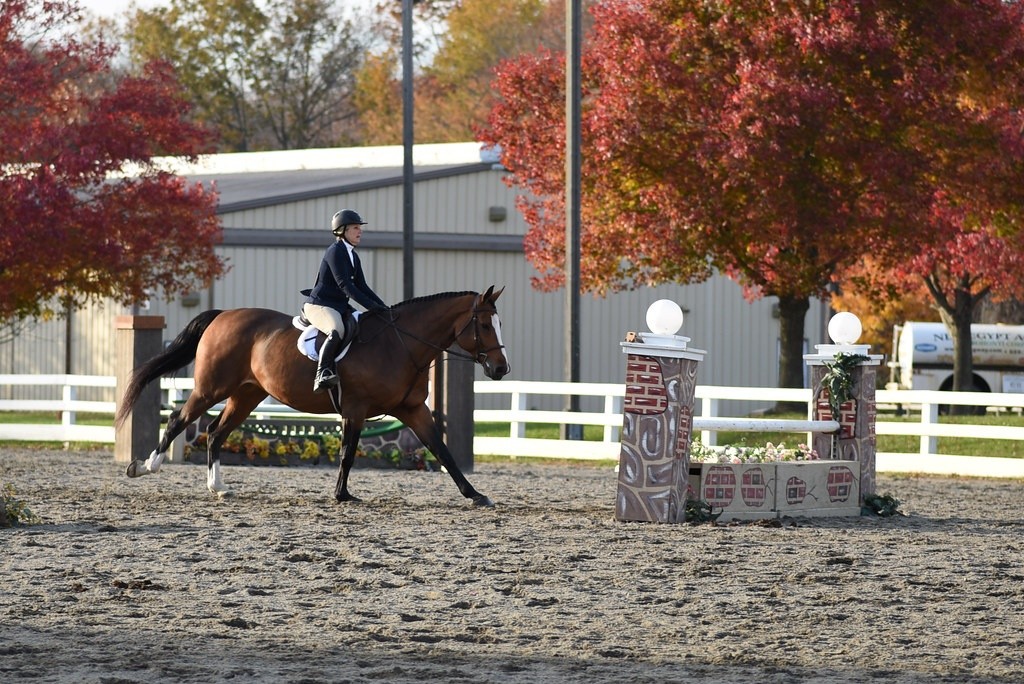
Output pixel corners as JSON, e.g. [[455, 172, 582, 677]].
[[690, 436, 820, 464]]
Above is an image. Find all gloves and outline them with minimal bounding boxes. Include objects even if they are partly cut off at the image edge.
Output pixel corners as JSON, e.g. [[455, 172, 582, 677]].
[[370, 303, 388, 313]]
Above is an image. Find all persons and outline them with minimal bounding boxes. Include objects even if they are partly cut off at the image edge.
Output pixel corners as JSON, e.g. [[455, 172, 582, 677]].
[[300, 211, 389, 394]]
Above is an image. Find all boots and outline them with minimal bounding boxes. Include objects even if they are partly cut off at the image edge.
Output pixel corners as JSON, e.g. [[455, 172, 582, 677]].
[[313, 329, 341, 394]]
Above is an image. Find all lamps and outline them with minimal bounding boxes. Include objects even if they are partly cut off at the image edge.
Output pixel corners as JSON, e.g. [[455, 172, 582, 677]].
[[645, 299, 684, 335], [827, 312, 863, 344]]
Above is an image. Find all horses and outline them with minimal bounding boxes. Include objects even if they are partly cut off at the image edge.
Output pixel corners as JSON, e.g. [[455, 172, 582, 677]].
[[118, 284, 510, 507]]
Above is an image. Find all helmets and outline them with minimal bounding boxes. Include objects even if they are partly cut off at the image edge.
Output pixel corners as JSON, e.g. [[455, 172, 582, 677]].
[[331, 209, 367, 235]]
